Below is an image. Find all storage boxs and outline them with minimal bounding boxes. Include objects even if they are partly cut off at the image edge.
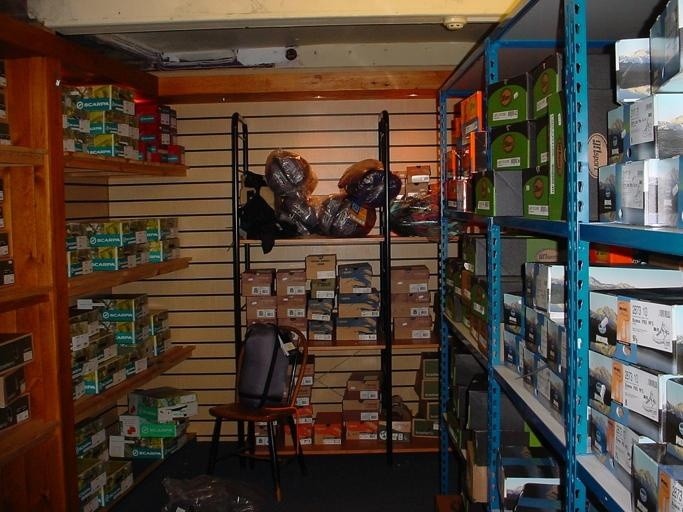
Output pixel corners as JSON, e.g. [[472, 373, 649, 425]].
[[238, 254, 442, 449]]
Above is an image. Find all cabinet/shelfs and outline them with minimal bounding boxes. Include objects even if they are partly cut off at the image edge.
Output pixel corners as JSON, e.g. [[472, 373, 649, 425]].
[[227, 108, 452, 458], [1, 80, 196, 511], [440, 1, 683, 510], [431, 2, 682, 511], [0, 15, 200, 512]]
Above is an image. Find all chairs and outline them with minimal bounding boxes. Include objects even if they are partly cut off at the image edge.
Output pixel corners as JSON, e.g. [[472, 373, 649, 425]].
[[204, 319, 310, 503]]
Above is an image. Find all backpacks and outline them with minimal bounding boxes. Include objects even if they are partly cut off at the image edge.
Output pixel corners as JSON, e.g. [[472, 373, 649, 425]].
[[236, 322, 293, 413]]
